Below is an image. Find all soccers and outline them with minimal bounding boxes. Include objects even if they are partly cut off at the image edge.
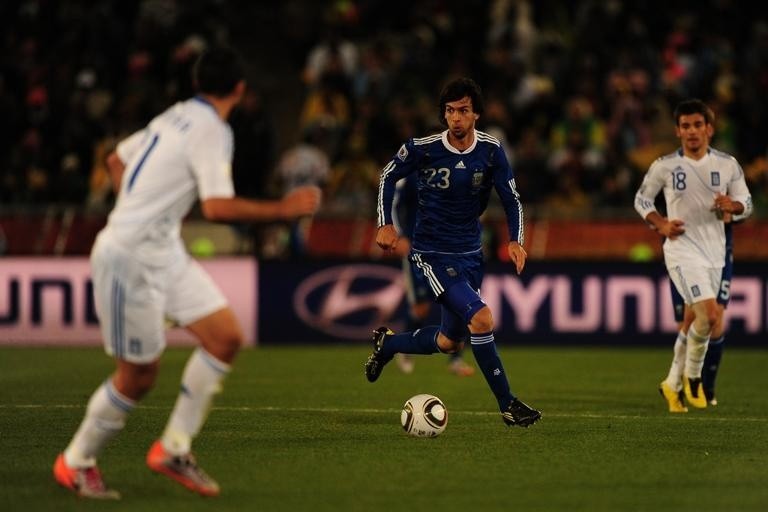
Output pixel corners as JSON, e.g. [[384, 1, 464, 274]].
[[400, 395, 448, 438]]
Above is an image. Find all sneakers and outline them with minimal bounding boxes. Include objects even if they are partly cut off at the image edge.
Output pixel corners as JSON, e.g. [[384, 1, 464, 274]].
[[680, 368, 707, 409], [147, 438, 222, 497], [448, 358, 475, 377], [659, 379, 689, 414], [53, 450, 122, 502], [397, 352, 415, 376], [500, 398, 542, 428], [365, 326, 396, 384]]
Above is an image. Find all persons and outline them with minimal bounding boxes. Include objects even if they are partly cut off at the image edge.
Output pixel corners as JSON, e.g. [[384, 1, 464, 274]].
[[0, 2, 768, 255], [365, 79, 543, 430], [631, 97, 754, 414], [53, 43, 324, 502], [653, 104, 733, 409]]
